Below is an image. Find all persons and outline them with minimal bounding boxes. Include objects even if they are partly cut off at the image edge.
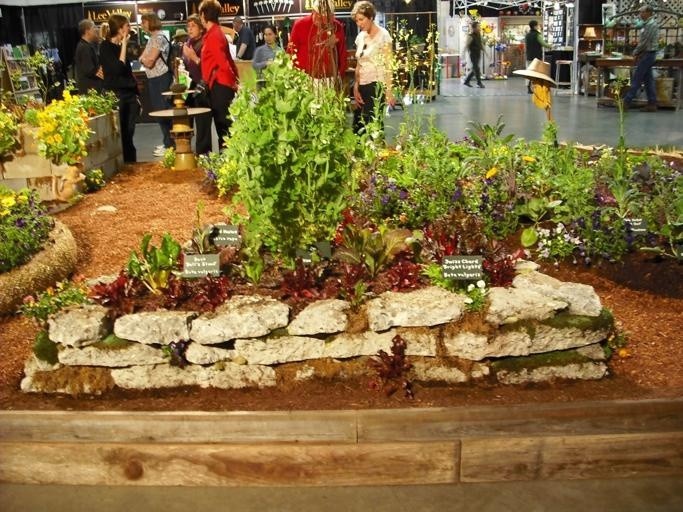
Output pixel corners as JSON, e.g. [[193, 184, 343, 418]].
[[351, 1, 397, 148], [619, 4, 659, 114], [461, 22, 488, 89], [283, 1, 348, 93], [523, 21, 552, 95], [73, 0, 285, 163]]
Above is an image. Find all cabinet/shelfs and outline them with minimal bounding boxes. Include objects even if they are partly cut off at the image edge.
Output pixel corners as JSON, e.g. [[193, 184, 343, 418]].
[[578, 37, 603, 98], [0, 44, 45, 106], [595, 57, 682, 112]]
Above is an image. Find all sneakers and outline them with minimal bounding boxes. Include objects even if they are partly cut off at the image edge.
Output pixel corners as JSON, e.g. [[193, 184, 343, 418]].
[[477, 82, 485, 88], [464, 81, 472, 87], [640, 103, 656, 112], [152, 145, 166, 156]]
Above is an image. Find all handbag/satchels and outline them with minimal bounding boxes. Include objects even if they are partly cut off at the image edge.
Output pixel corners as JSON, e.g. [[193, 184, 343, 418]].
[[190, 80, 212, 109], [169, 41, 182, 68]]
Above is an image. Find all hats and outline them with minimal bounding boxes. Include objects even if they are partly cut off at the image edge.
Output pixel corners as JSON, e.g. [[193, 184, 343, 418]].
[[513, 58, 558, 88], [172, 29, 188, 38]]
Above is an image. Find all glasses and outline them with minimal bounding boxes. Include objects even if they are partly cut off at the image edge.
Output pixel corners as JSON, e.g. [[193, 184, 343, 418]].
[[360, 44, 366, 57]]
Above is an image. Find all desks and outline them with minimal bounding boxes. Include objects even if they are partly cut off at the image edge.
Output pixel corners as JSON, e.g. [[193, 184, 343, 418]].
[[554, 60, 572, 95]]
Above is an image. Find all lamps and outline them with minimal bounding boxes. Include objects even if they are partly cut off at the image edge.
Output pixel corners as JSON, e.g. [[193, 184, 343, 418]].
[[582, 25, 598, 52]]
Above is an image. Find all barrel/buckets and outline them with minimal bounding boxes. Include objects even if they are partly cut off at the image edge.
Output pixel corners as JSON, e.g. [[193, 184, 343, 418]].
[[656, 77, 675, 101]]
